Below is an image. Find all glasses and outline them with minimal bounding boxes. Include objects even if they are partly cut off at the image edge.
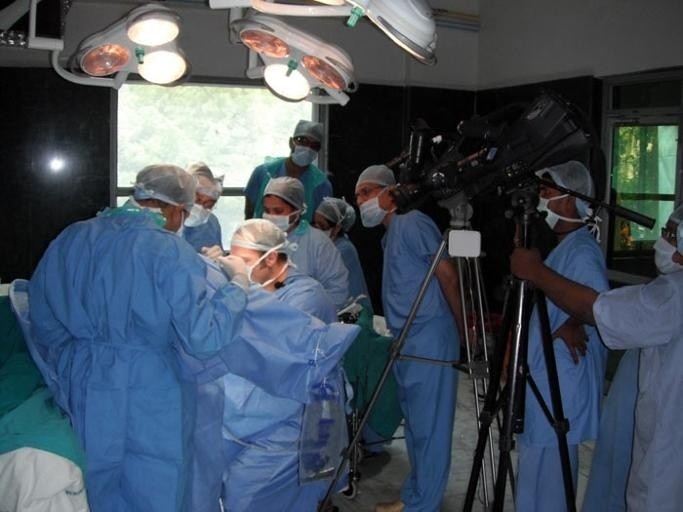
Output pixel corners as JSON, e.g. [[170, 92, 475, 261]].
[[661, 227, 676, 240], [293, 136, 322, 152]]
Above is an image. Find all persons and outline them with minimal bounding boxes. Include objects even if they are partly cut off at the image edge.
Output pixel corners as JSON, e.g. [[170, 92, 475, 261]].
[[313, 197, 386, 455], [356, 164, 465, 512], [201, 218, 350, 511], [509, 204, 683, 512], [244, 120, 332, 226], [514, 162, 609, 512], [181, 165, 223, 254], [27, 167, 249, 512], [260, 176, 350, 308]]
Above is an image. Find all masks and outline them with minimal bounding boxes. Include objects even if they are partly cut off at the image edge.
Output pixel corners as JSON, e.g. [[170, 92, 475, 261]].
[[359, 187, 397, 227], [184, 203, 211, 227], [291, 146, 317, 167], [246, 246, 289, 287], [262, 209, 301, 232], [537, 194, 583, 230], [652, 237, 683, 274], [323, 228, 332, 238]]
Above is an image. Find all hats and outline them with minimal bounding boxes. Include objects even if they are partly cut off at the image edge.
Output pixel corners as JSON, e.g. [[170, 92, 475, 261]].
[[670, 204, 683, 223], [186, 160, 225, 200], [293, 119, 324, 141], [230, 218, 300, 255], [263, 176, 304, 209], [356, 165, 396, 187], [134, 163, 197, 213], [535, 160, 595, 218], [316, 196, 356, 232]]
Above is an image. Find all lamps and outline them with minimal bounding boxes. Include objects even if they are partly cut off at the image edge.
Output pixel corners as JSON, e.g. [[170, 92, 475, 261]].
[[312, 1, 442, 69], [76, 5, 193, 89], [228, 15, 355, 105]]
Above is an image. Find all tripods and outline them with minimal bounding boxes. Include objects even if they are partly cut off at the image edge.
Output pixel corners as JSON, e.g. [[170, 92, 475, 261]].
[[461, 188, 579, 510], [320, 198, 496, 512]]
